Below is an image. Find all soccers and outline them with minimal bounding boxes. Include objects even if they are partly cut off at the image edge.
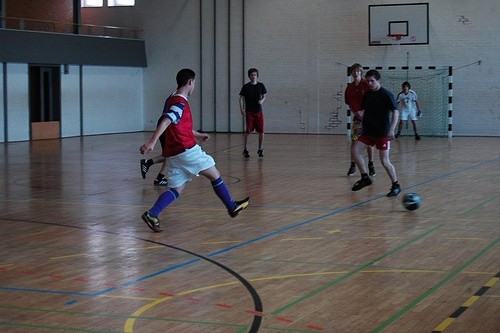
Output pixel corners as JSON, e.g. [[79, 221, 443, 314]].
[[402, 193, 422, 210]]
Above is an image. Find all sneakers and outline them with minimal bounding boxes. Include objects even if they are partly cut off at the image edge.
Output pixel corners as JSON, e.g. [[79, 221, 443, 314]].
[[153, 178, 168, 185], [369, 166, 377, 177], [387, 184, 402, 197], [352, 178, 372, 191], [243, 150, 250, 158], [141, 159, 149, 179], [228, 197, 250, 218], [349, 167, 356, 176], [142, 211, 163, 232], [257, 149, 263, 157]]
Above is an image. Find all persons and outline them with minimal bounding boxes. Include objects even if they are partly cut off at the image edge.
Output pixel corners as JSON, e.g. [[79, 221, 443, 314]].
[[352, 69, 402, 197], [141, 153, 168, 186], [344, 63, 376, 176], [140, 69, 250, 233], [395, 81, 422, 140], [239, 68, 267, 158]]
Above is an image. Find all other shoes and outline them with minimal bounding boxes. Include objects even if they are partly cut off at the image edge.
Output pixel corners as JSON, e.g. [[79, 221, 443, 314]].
[[395, 133, 401, 137], [415, 135, 421, 140]]
